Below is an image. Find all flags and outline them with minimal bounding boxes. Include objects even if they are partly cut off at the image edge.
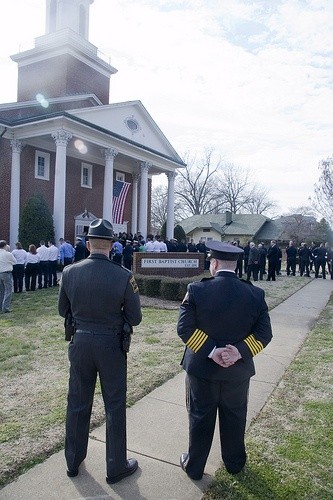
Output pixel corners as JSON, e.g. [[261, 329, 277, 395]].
[[112, 180, 131, 224]]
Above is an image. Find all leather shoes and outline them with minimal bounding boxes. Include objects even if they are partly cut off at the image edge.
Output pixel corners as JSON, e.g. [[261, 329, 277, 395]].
[[181, 453, 190, 472], [66, 464, 78, 478], [106, 458, 139, 483]]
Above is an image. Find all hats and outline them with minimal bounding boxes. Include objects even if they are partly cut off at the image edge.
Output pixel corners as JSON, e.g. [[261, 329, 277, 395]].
[[204, 239, 245, 261], [78, 218, 117, 240]]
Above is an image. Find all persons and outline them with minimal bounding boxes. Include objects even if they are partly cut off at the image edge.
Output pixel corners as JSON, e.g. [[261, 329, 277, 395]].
[[57, 218, 138, 484], [0, 230, 333, 315], [176, 239, 274, 481]]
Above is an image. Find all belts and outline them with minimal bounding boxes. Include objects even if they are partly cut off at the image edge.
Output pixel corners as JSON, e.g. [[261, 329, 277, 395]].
[[75, 329, 119, 335]]
